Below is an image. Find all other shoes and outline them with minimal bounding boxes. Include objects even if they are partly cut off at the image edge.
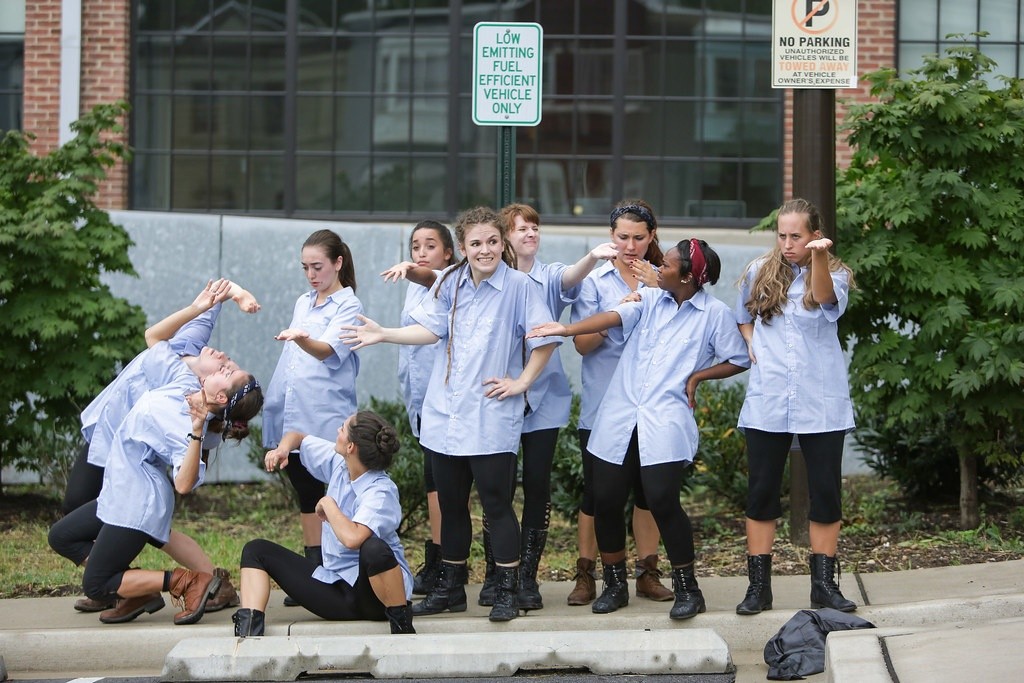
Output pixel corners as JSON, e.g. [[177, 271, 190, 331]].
[[74, 598, 116, 612], [204, 569, 240, 612]]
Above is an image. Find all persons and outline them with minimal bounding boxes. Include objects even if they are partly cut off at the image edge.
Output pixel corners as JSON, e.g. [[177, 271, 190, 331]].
[[232, 410, 417, 637], [261, 229, 367, 606], [338, 199, 750, 621], [735, 198, 859, 615], [46, 278, 265, 624]]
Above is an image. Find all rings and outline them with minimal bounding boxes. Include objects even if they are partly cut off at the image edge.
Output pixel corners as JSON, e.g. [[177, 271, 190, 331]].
[[216, 291, 219, 294]]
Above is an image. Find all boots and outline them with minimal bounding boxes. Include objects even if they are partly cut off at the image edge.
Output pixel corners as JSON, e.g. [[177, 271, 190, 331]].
[[736, 554, 773, 615], [284, 545, 323, 606], [567, 558, 597, 606], [517, 528, 548, 609], [99, 590, 165, 622], [386, 600, 416, 634], [478, 526, 499, 605], [232, 609, 266, 637], [669, 563, 706, 619], [412, 540, 443, 595], [635, 554, 676, 601], [410, 562, 468, 615], [809, 553, 857, 612], [489, 564, 520, 621], [169, 566, 222, 625], [591, 559, 629, 612]]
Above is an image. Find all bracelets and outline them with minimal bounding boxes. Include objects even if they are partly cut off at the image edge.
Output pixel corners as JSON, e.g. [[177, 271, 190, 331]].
[[599, 332, 607, 338]]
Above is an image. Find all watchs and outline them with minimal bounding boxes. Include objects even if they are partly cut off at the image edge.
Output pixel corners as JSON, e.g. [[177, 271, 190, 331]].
[[186, 433, 204, 442]]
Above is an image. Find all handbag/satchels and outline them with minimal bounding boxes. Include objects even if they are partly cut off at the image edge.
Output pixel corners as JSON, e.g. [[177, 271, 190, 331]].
[[763, 609, 877, 680]]
